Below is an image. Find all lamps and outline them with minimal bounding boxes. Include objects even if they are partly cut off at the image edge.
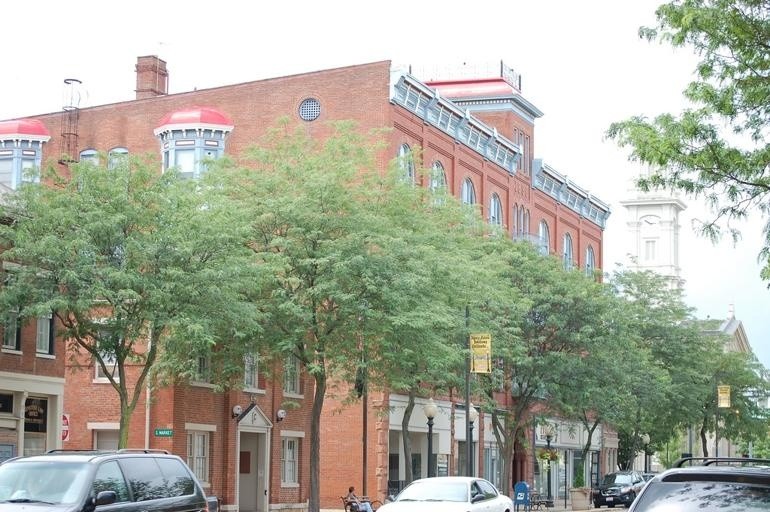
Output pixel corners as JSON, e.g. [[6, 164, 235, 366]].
[[277, 409, 286, 423], [232, 405, 242, 418]]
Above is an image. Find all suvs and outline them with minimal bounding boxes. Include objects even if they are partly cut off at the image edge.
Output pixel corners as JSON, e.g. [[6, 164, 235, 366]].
[[590, 451, 770, 512], [0, 444, 223, 512]]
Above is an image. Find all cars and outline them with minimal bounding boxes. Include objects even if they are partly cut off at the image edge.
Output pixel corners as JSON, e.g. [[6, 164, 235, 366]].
[[371, 475, 517, 512]]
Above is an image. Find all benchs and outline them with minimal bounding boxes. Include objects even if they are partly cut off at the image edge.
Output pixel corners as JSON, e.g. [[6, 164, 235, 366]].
[[340, 495, 384, 512], [530, 493, 554, 511]]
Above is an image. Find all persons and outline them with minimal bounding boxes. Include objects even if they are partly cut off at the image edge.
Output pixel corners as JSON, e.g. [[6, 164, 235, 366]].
[[346, 486, 374, 512]]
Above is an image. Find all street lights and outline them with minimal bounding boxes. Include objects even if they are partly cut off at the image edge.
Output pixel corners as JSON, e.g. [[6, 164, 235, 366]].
[[642, 432, 652, 473], [545, 423, 556, 508], [468, 401, 478, 477], [422, 394, 438, 478]]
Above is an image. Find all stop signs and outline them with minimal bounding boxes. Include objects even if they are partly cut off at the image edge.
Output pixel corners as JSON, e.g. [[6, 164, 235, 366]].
[[61, 413, 70, 442]]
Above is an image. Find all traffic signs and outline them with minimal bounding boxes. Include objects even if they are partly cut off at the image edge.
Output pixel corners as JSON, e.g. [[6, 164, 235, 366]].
[[154, 429, 173, 437]]
